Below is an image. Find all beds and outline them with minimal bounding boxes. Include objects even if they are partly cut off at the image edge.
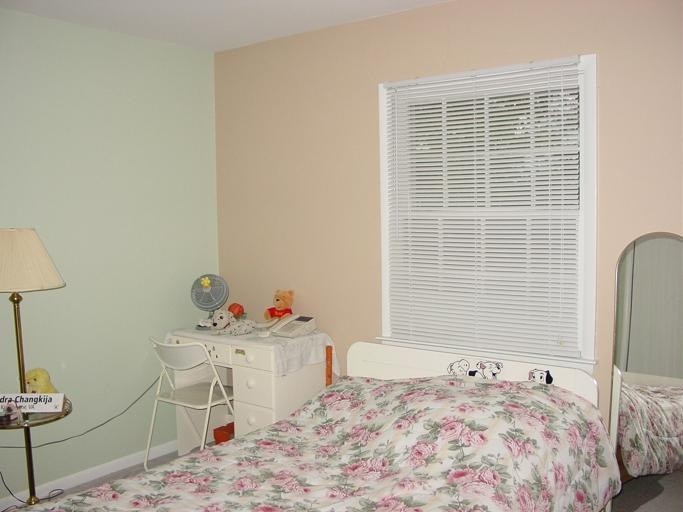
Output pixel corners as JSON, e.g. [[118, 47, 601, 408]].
[[609, 363, 683, 483], [11, 341, 602, 512]]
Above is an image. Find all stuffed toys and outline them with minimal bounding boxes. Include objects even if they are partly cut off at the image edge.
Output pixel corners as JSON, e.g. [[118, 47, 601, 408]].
[[213, 310, 278, 335], [27, 368, 56, 395], [263, 289, 293, 320]]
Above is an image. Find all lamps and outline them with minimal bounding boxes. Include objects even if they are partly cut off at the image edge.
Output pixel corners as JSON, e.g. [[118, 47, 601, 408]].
[[0, 229, 72, 504]]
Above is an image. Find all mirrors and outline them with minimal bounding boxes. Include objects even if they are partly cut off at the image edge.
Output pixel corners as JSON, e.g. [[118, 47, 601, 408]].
[[605, 231, 683, 510]]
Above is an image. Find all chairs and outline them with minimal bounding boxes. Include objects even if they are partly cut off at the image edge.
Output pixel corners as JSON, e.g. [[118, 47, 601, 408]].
[[143, 337, 233, 471]]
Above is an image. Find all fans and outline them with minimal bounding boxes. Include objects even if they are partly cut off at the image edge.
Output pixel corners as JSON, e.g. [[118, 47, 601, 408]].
[[189, 274, 228, 332]]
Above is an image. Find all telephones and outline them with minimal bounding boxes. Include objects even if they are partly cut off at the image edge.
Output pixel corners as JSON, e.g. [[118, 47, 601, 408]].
[[270, 313, 316, 338]]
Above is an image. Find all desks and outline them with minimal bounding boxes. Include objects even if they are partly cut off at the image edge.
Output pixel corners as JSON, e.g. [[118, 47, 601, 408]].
[[172, 328, 332, 457]]
